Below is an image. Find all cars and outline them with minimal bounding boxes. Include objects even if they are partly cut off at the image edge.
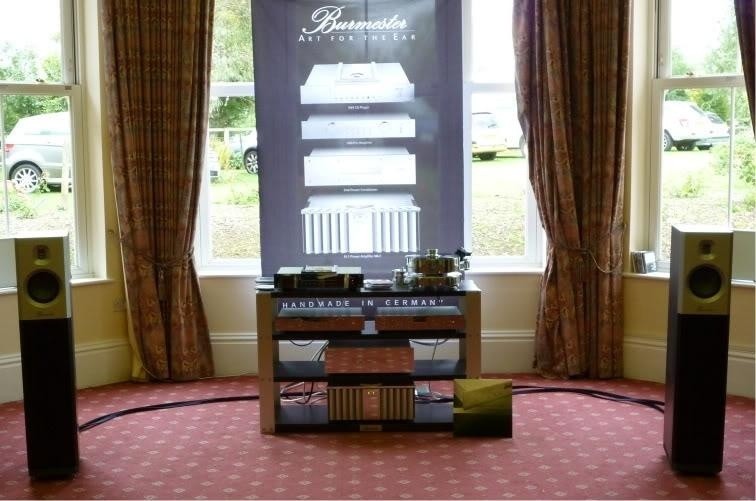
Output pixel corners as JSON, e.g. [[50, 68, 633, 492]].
[[662, 100, 730, 152], [471, 101, 528, 164], [3, 111, 74, 195]]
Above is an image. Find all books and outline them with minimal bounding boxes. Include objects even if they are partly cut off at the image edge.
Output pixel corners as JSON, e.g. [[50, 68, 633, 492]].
[[364, 284, 392, 292], [368, 280, 393, 287]]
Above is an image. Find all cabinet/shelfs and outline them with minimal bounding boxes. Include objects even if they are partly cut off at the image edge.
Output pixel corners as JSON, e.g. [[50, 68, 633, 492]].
[[254, 281, 482, 437]]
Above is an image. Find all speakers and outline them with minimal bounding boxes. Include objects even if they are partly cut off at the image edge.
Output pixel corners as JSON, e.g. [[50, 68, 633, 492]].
[[662, 220, 734, 475], [14, 229, 80, 483]]
